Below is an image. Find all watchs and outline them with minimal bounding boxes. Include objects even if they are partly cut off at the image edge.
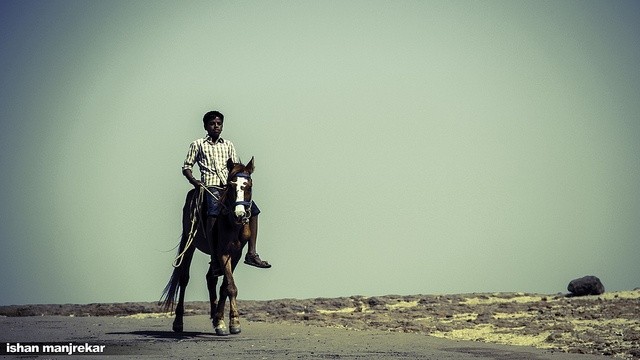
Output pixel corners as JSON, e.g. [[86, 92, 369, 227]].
[[188, 178, 196, 183]]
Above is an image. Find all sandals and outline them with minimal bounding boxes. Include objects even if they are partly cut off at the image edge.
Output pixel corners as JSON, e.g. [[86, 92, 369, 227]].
[[243, 253, 271, 268], [209, 260, 222, 277]]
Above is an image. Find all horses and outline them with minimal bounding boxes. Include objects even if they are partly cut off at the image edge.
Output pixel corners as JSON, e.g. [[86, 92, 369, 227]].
[[158, 156, 255, 336]]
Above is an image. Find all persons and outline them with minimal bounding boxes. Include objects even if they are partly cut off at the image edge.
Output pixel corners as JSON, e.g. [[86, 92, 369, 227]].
[[182, 111, 272, 277]]
